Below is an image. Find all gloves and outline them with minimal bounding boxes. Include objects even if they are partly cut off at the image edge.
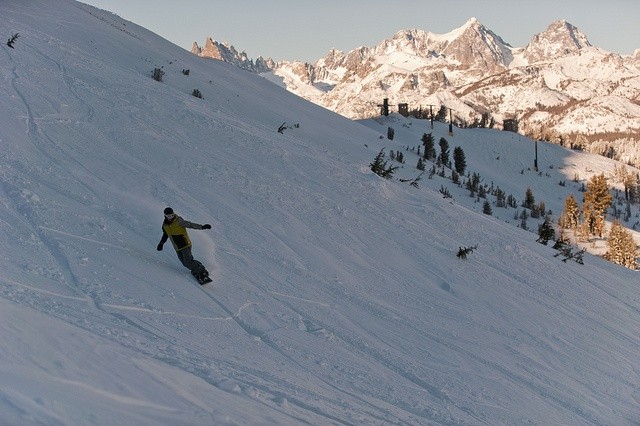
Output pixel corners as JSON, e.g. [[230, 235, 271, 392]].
[[201, 224, 211, 229], [158, 243, 163, 250]]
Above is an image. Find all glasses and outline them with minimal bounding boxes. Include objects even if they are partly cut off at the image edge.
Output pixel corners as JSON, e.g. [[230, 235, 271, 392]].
[[165, 214, 175, 217]]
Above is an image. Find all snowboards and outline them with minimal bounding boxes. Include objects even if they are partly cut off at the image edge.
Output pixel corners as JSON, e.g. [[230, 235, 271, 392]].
[[191, 270, 213, 285]]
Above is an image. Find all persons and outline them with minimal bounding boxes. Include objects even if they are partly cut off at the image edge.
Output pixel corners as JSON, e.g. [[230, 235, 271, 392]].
[[156, 206, 212, 280]]
